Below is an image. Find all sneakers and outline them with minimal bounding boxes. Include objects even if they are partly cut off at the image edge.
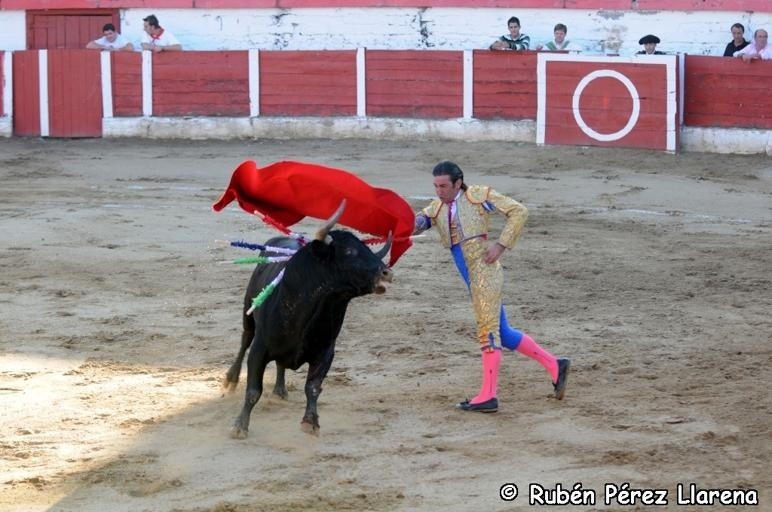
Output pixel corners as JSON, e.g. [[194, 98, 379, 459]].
[[456, 397, 499, 412], [552, 358, 571, 400]]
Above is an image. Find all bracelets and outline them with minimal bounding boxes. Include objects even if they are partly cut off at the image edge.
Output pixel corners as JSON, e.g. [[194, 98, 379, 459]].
[[161, 45, 165, 51]]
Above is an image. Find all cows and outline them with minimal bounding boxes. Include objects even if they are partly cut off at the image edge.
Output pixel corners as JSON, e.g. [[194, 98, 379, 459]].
[[219, 199, 394, 440]]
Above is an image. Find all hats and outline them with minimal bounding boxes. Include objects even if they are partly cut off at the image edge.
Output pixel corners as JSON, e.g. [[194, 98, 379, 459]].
[[639, 34, 660, 44]]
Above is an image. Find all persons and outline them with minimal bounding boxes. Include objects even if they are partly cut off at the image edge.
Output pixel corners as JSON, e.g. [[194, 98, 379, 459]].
[[732, 30, 771, 63], [723, 23, 751, 57], [140, 14, 183, 52], [85, 23, 134, 52], [634, 35, 667, 55], [537, 24, 582, 51], [411, 161, 571, 413], [488, 17, 530, 50]]
